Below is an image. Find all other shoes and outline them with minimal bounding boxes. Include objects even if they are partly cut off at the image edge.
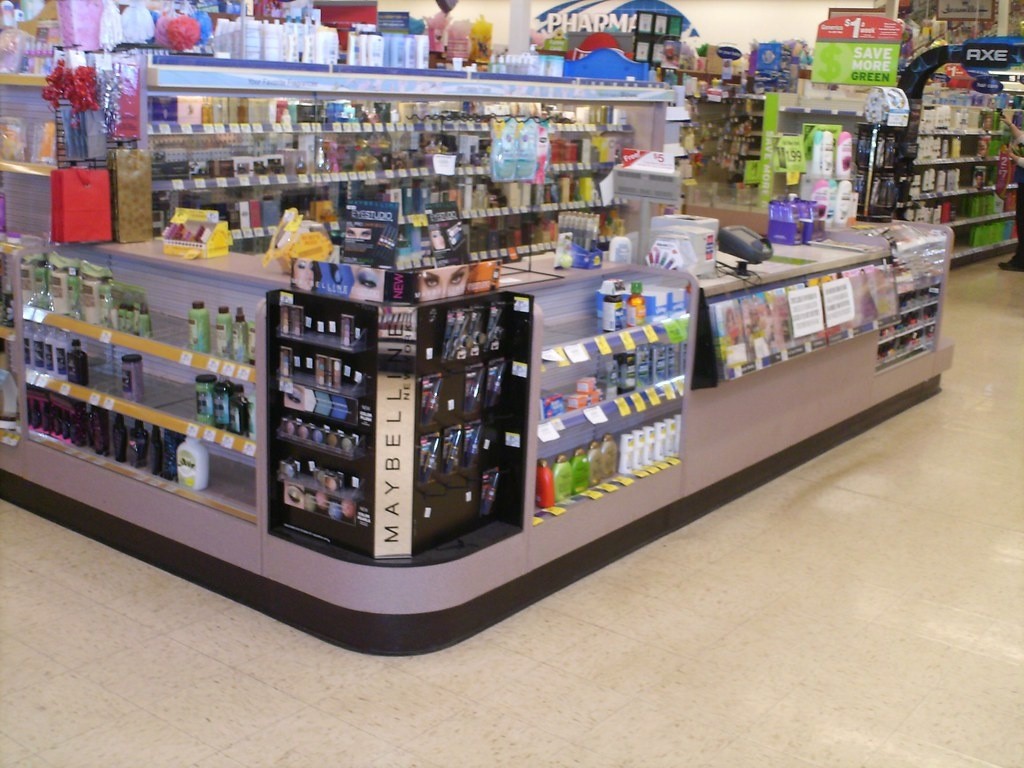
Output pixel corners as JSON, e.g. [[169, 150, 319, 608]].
[[998, 257, 1023, 272]]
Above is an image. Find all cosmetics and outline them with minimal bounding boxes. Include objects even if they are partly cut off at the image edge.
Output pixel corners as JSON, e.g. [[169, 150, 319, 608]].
[[1, 0, 1023, 563]]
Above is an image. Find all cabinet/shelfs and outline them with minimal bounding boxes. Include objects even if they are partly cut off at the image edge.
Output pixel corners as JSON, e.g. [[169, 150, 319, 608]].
[[904, 105, 1021, 267], [4, 70, 116, 414], [0, 238, 704, 570], [140, 55, 683, 273], [11, 211, 958, 658], [871, 224, 946, 368]]
